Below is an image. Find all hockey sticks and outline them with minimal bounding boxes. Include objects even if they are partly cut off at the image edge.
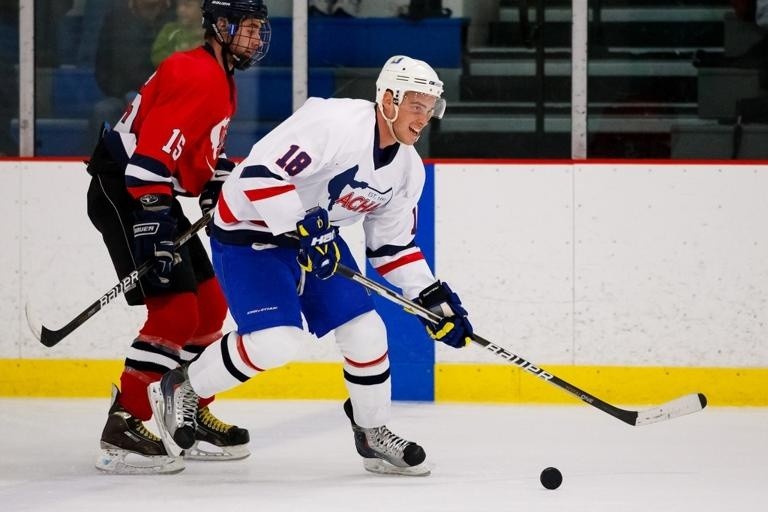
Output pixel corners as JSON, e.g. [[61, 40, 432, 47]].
[[296, 251, 706, 426], [25, 208, 215, 347]]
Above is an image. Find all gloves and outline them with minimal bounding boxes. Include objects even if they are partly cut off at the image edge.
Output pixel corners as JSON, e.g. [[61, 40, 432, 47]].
[[199, 158, 235, 237], [412, 280, 472, 348], [133, 194, 181, 283], [297, 206, 340, 281]]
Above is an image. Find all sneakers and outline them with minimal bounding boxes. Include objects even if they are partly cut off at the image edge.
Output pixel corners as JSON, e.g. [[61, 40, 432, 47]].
[[344, 398, 426, 467], [161, 365, 199, 449], [101, 382, 184, 456], [194, 408, 249, 446]]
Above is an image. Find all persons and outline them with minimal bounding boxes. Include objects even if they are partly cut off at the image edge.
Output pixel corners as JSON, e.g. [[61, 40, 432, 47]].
[[88, 1, 173, 125], [151, 0, 206, 71], [160, 51, 474, 470], [85, 1, 275, 458]]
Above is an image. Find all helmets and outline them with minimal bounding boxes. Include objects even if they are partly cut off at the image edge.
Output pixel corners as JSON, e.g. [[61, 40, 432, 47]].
[[201, 0, 271, 70], [376, 55, 446, 120]]
